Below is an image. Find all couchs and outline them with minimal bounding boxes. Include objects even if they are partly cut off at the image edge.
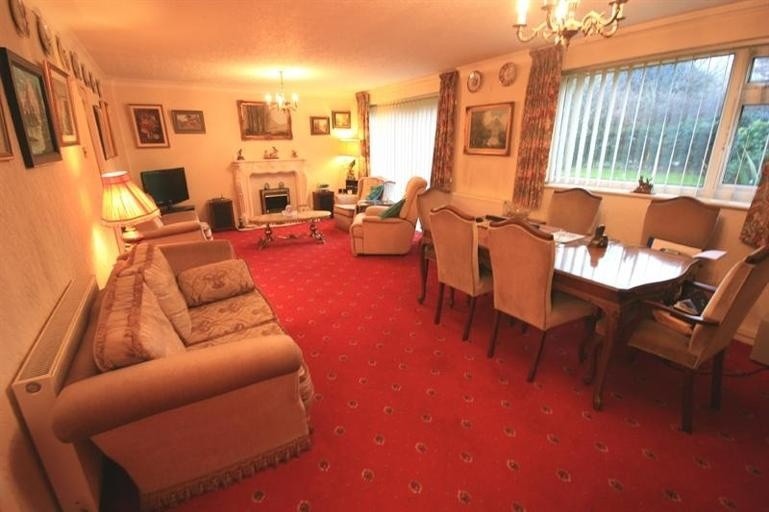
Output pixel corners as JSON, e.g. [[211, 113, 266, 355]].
[[53, 240, 314, 512]]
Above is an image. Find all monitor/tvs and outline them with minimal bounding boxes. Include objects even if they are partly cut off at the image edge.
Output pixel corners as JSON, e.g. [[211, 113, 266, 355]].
[[140, 167, 195, 212]]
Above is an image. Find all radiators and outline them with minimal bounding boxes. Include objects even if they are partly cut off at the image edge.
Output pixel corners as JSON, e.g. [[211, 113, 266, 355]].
[[11, 274, 104, 512]]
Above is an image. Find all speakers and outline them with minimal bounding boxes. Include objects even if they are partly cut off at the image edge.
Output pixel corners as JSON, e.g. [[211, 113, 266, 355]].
[[312, 191, 334, 218], [207, 198, 235, 231]]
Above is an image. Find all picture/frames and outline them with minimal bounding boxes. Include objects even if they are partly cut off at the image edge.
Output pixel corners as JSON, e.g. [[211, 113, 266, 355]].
[[127, 104, 170, 149], [0, 0, 118, 169], [332, 111, 351, 128], [237, 100, 293, 141], [171, 110, 206, 134], [310, 116, 330, 135], [464, 101, 514, 156]]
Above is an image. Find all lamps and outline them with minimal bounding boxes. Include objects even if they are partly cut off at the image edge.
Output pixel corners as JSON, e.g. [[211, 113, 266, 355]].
[[264, 71, 299, 111], [512, 0, 628, 51], [100, 171, 161, 251]]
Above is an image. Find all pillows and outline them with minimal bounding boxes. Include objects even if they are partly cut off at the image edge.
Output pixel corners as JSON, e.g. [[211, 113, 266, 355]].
[[378, 199, 405, 218], [94, 245, 255, 372], [366, 185, 384, 200]]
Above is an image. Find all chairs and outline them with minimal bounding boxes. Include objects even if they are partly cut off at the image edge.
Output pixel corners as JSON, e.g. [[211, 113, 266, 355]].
[[582, 243, 769, 432], [427, 205, 513, 342], [415, 187, 473, 308], [484, 217, 599, 386], [349, 177, 427, 257], [333, 177, 384, 233], [579, 194, 724, 356], [121, 211, 213, 245], [509, 187, 604, 334]]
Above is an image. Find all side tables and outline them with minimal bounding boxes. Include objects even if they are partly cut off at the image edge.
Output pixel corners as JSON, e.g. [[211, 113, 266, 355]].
[[356, 200, 394, 214]]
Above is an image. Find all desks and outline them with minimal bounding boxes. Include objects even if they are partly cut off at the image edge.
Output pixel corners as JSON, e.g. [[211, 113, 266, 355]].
[[478, 212, 706, 414]]
